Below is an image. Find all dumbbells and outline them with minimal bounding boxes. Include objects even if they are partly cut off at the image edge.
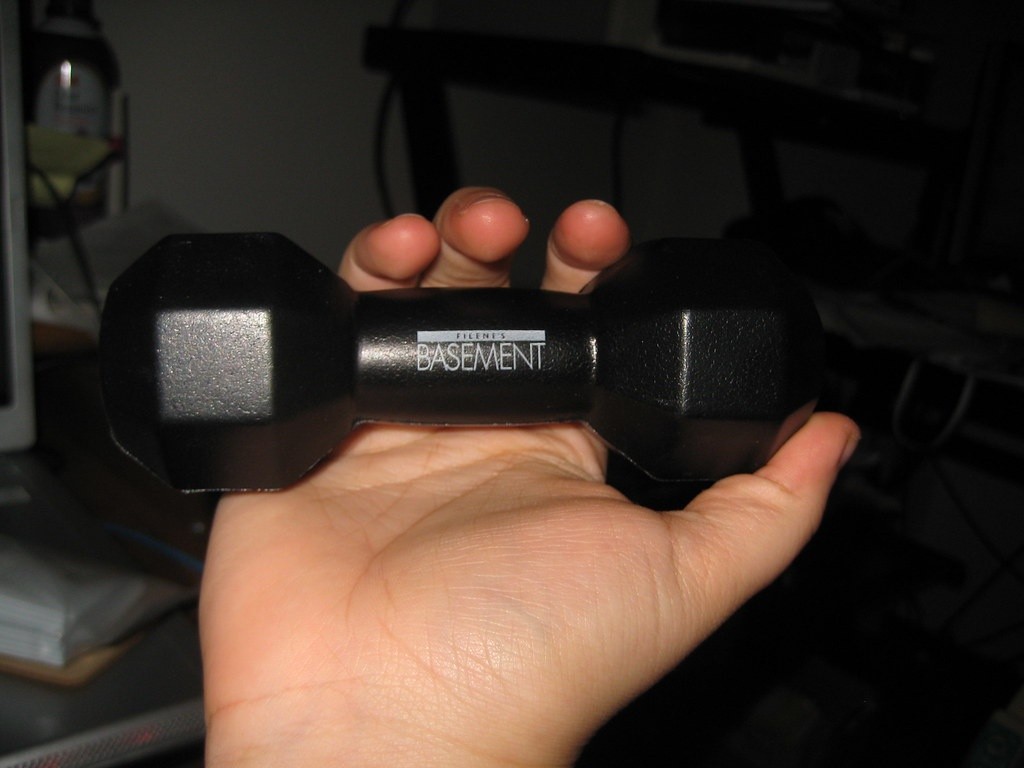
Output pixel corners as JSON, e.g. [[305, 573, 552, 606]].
[[98, 230, 819, 494]]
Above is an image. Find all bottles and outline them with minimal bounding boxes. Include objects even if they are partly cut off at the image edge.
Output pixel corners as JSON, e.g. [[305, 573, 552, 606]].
[[21, 0, 118, 233]]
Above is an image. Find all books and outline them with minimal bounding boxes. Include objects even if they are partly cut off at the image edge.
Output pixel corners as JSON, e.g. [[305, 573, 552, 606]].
[[0, 535, 198, 666]]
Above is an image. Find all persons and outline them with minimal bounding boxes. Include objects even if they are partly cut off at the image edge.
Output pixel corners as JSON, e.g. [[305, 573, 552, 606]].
[[198, 186, 861, 768]]
